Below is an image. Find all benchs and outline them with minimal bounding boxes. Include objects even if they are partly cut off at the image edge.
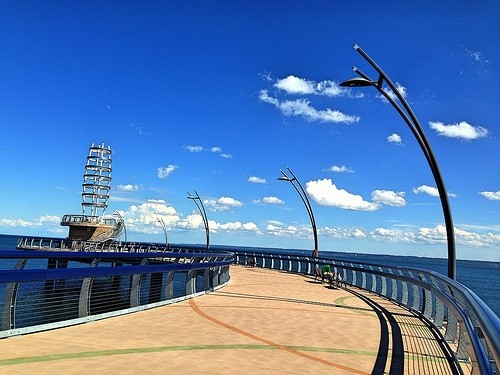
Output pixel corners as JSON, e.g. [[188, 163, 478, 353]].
[[313, 262, 348, 290], [246, 258, 260, 268]]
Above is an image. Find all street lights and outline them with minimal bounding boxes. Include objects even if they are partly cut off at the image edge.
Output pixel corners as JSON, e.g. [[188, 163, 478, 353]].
[[186, 189, 209, 249], [339, 43, 457, 280], [155, 217, 168, 248], [278, 166, 319, 260], [113, 210, 127, 246]]
